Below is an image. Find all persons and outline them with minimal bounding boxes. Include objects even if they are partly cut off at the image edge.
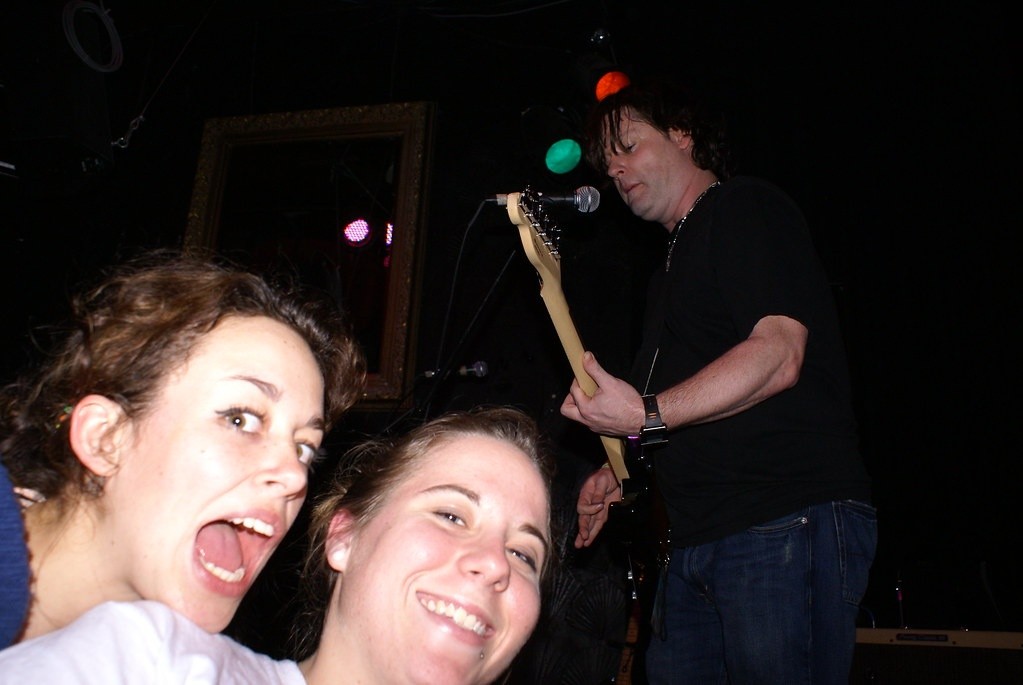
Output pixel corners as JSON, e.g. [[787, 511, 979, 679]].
[[0, 248, 366, 649], [0, 403, 552, 685], [559, 81, 879, 685]]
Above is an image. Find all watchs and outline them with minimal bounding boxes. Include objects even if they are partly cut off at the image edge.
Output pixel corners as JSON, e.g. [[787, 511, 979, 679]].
[[639, 394, 668, 446]]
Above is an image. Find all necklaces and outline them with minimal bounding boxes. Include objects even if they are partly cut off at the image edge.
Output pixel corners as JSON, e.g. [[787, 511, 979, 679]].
[[665, 181, 719, 272]]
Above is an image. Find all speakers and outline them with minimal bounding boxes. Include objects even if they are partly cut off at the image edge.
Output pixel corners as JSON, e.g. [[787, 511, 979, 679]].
[[847, 627, 1022, 685]]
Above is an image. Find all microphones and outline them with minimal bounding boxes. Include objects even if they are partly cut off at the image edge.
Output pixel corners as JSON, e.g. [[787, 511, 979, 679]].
[[421, 362, 488, 378], [485, 186, 600, 212]]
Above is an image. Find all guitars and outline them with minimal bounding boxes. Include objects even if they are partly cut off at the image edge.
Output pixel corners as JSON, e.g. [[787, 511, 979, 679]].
[[506, 183, 667, 622]]
